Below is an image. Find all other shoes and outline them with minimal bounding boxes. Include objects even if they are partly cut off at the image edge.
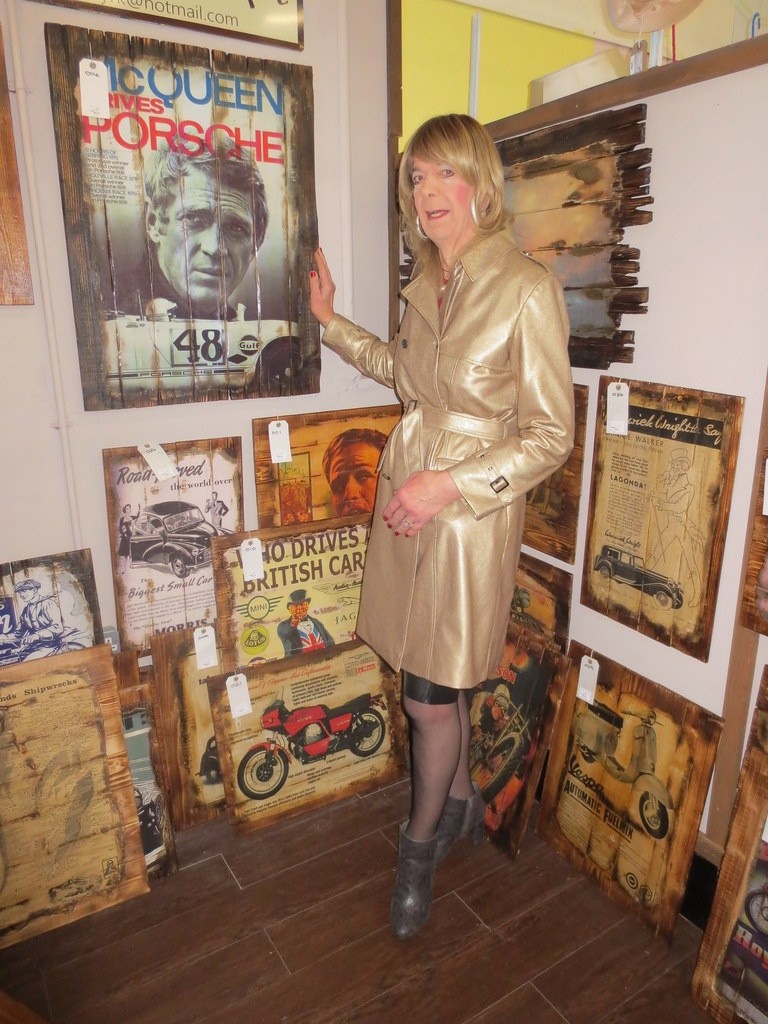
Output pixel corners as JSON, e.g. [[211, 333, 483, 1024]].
[[137, 800, 166, 866]]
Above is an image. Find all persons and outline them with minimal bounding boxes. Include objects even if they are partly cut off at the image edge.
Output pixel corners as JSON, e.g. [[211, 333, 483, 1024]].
[[309, 114, 575, 939], [117, 129, 269, 322], [321, 428, 388, 517]]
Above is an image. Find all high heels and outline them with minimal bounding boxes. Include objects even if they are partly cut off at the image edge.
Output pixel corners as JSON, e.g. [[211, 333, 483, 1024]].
[[391, 819, 439, 940], [436, 781, 486, 863]]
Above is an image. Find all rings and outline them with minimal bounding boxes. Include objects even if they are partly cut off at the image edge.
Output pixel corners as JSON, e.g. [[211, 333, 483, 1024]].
[[403, 518, 411, 526]]
